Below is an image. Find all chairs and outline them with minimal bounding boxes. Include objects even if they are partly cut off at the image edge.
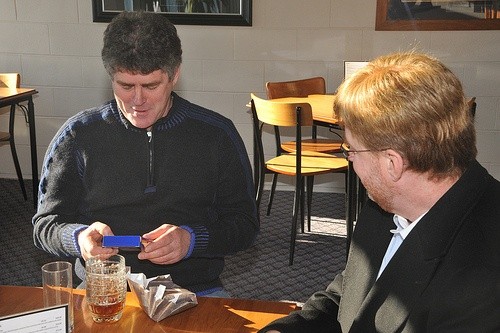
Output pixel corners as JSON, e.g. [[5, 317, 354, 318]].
[[246, 90, 355, 266], [0, 71, 28, 200], [265, 74, 356, 233]]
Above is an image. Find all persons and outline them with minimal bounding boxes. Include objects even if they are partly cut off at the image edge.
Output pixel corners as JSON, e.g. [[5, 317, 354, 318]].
[[252, 52, 500, 333], [31, 10, 261, 300]]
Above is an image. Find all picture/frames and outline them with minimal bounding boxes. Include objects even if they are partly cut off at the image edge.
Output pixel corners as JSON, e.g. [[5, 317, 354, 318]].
[[91, 0, 253, 26], [374, 0, 500, 31]]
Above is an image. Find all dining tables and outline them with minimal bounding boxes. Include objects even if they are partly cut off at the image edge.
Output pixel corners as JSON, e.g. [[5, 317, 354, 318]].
[[0, 285, 306, 333]]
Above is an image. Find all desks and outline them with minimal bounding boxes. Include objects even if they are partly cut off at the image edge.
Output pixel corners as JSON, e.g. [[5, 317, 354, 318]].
[[246, 93, 477, 263], [0, 86, 40, 208]]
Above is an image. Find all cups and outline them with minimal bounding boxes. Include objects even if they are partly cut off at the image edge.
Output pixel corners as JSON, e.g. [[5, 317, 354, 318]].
[[85, 253, 126, 324], [41, 262, 75, 333]]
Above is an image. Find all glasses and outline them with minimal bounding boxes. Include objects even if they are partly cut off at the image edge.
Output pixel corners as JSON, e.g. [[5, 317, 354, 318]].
[[340, 141, 393, 162]]
